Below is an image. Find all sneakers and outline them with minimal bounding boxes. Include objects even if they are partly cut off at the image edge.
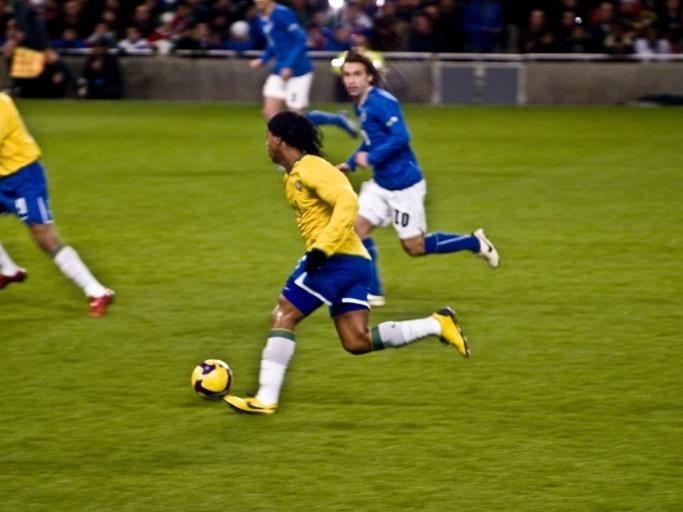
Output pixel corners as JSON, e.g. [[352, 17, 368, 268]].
[[224, 395, 278, 416], [87, 288, 115, 316], [0, 268, 26, 291], [474, 228, 499, 268], [367, 292, 386, 307], [433, 307, 469, 357]]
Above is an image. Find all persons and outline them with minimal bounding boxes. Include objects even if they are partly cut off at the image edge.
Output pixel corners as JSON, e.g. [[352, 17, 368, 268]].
[[0, 91, 115, 311], [332, 51, 500, 268], [355, 1, 683, 56], [247, 0, 359, 171], [223, 113, 471, 414], [0, 1, 246, 96]]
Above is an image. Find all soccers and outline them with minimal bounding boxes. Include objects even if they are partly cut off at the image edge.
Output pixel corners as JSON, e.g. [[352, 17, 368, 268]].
[[191, 358, 232, 398]]
